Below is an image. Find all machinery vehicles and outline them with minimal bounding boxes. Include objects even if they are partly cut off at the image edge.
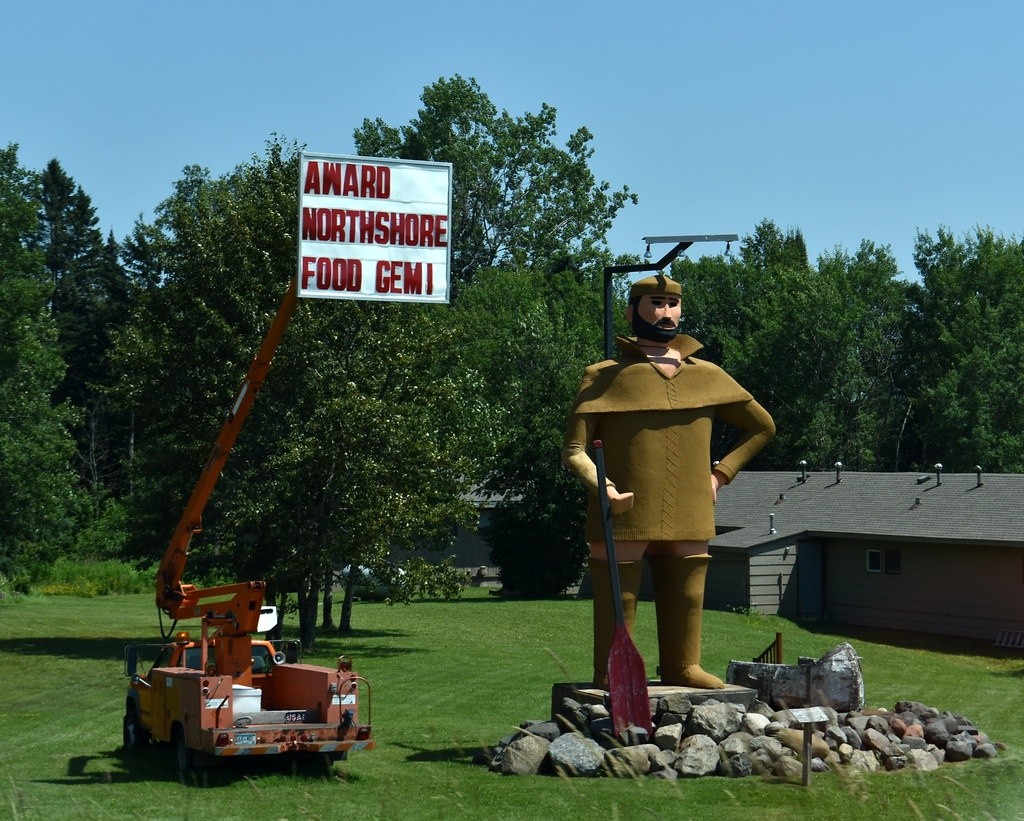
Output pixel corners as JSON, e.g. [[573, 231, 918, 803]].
[[122, 153, 452, 778]]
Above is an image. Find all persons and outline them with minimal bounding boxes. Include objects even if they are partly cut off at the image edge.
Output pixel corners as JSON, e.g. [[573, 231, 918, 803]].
[[562, 275, 775, 693]]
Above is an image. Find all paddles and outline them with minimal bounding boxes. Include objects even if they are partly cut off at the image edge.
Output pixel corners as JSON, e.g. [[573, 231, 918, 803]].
[[593, 438, 652, 739]]
